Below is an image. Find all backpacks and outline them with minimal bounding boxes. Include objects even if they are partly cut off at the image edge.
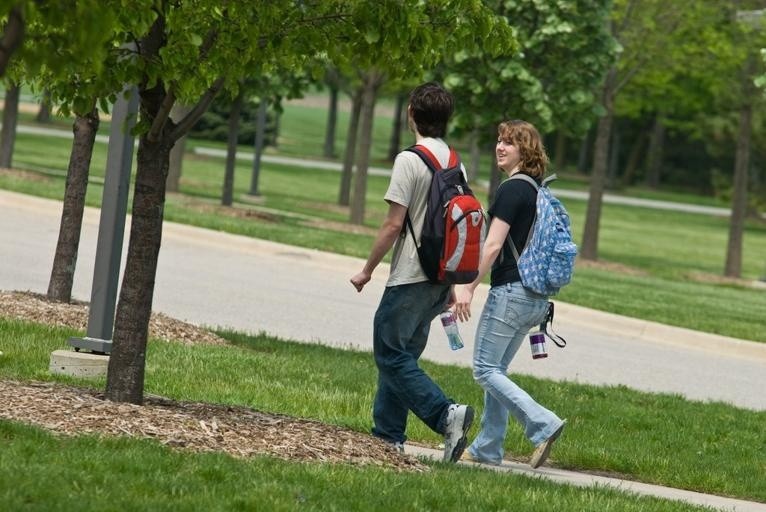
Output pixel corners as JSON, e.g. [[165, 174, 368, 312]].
[[405, 141, 489, 286], [497, 176, 577, 298]]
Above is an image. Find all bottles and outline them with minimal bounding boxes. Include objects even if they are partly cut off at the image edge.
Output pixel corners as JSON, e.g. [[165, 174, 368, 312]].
[[439, 308, 464, 353], [528, 330, 547, 360]]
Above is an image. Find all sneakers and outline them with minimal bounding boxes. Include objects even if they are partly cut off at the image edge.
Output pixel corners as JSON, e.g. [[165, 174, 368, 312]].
[[457, 447, 504, 467], [441, 401, 475, 470], [528, 417, 565, 472]]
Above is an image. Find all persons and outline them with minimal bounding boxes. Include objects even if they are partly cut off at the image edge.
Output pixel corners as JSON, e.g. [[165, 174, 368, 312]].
[[350, 83, 475, 466], [453, 118, 565, 470]]
